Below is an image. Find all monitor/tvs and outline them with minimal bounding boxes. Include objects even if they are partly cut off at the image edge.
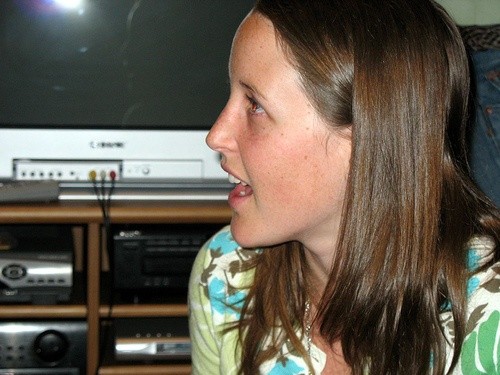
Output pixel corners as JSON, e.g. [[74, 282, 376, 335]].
[[0, 0, 256, 200]]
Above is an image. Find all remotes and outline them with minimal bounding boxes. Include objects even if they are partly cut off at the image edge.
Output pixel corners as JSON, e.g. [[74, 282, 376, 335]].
[[1, 179, 60, 202]]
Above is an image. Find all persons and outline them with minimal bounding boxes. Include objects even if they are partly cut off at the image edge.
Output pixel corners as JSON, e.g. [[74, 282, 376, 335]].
[[186, 0, 500, 374]]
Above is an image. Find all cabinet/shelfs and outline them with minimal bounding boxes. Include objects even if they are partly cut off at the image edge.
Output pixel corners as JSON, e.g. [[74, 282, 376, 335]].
[[0, 204, 233, 375]]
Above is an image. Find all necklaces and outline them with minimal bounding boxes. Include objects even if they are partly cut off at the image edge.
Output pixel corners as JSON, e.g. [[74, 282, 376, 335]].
[[302, 298, 314, 358]]
